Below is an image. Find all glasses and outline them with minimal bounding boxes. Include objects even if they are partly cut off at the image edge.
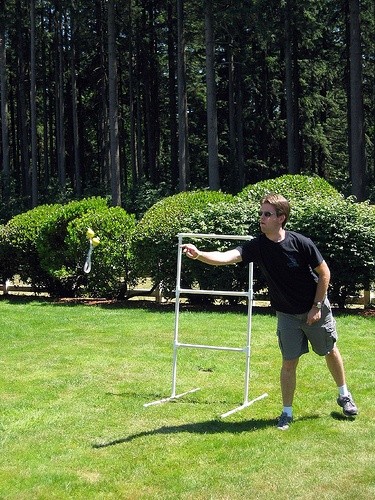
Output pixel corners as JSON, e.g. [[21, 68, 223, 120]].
[[257, 211, 277, 217]]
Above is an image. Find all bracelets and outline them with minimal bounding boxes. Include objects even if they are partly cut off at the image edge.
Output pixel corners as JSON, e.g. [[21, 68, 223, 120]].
[[192, 250, 200, 260]]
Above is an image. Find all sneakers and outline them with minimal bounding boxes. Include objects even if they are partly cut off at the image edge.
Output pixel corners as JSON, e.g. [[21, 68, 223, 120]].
[[277, 413, 293, 431], [337, 392, 358, 415]]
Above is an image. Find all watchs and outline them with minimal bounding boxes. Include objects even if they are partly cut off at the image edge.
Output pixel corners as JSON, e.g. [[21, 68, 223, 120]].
[[312, 301, 322, 309]]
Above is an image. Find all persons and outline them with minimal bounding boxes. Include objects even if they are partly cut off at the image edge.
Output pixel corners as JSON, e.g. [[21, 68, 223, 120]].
[[179, 194, 358, 432]]
